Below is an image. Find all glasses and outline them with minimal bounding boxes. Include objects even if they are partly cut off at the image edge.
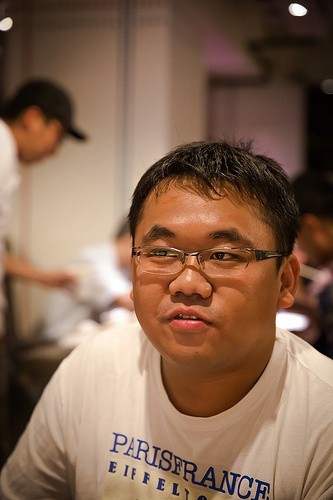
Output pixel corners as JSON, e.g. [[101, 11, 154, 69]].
[[131, 245, 292, 277]]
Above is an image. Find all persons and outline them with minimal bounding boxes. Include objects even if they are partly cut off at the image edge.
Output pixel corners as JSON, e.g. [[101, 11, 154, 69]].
[[31, 197, 333, 345], [0, 139, 333, 500], [0, 76, 90, 466]]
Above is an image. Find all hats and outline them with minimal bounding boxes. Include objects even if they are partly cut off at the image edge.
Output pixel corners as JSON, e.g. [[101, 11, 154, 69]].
[[7, 77, 90, 141]]
